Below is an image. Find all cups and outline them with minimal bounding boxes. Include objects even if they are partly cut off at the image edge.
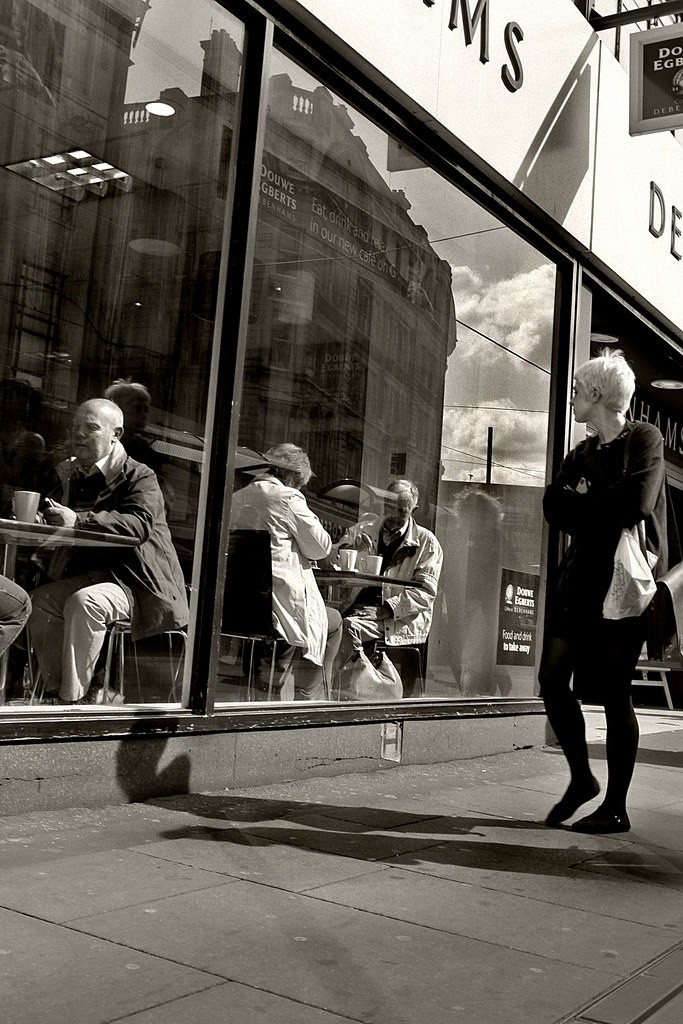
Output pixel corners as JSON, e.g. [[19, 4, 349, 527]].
[[339, 550, 358, 571], [361, 556, 383, 575], [12, 491, 41, 521]]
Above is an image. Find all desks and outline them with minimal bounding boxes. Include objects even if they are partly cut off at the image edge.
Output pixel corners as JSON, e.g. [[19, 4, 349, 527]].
[[0, 518, 141, 705], [311, 569, 423, 609]]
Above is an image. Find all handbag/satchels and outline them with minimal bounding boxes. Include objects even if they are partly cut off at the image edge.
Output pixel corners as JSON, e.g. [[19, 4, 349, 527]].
[[603, 520, 659, 619], [645, 559, 683, 661], [333, 648, 403, 701]]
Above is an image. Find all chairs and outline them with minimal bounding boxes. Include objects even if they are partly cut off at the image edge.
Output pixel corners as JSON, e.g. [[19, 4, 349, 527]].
[[0, 504, 427, 704]]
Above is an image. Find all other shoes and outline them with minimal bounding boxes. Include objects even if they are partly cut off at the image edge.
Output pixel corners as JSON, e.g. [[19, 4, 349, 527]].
[[546, 778, 600, 826], [572, 805, 631, 834]]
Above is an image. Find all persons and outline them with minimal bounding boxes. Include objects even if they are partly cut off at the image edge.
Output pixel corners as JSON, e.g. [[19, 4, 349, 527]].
[[0, 570, 32, 661], [438, 492, 515, 696], [538, 345, 670, 834], [227, 444, 344, 699], [101, 378, 177, 524], [27, 398, 190, 704], [334, 479, 444, 691]]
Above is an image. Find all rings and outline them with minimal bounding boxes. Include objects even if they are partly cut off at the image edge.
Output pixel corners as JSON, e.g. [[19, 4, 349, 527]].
[[52, 522, 54, 525]]
